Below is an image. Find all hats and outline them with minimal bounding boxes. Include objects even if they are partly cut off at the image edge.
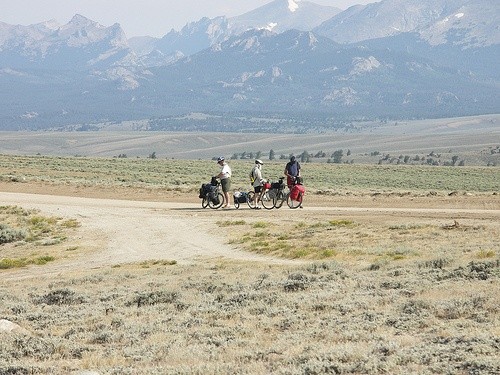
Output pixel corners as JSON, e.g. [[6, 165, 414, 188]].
[[217, 156, 225, 163], [255, 159, 263, 164]]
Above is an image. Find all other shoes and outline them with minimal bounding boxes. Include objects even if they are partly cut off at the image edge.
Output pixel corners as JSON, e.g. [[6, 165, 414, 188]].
[[221, 205, 231, 208], [254, 206, 261, 209]]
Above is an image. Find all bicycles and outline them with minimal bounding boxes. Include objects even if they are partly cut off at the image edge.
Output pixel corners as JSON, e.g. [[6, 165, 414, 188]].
[[201, 177, 225, 209], [232, 176, 304, 210]]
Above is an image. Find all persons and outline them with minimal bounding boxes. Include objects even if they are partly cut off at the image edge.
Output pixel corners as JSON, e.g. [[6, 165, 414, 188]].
[[214, 156, 231, 207], [250, 159, 264, 209], [284, 154, 303, 208]]
